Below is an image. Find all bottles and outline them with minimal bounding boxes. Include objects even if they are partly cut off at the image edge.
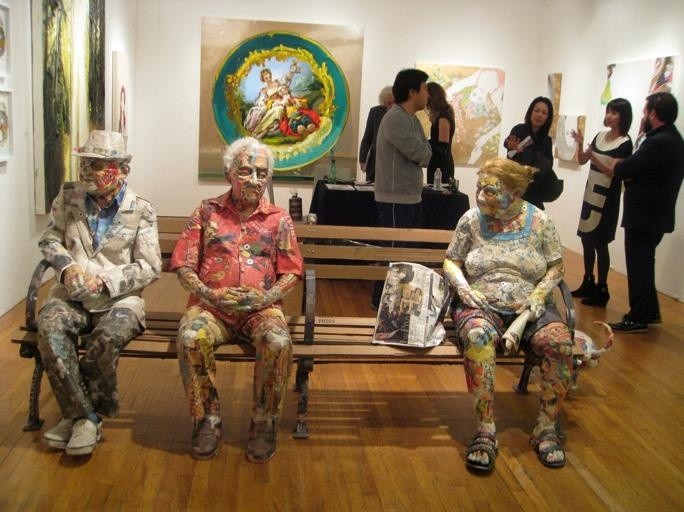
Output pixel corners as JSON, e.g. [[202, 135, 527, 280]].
[[328, 159, 337, 185], [433, 167, 442, 191], [289, 196, 302, 219]]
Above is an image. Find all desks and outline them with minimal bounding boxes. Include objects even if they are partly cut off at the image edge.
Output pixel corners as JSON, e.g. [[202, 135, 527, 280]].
[[309, 181, 469, 230]]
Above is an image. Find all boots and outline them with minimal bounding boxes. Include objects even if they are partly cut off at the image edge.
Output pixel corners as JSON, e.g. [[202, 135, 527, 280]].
[[581, 282, 610, 307], [571, 273, 597, 297]]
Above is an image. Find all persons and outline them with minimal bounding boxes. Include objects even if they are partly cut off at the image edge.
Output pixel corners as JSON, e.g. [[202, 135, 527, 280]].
[[371, 68, 432, 310], [446, 68, 505, 146], [244, 58, 297, 138], [443, 158, 574, 472], [468, 147, 482, 165], [557, 116, 578, 160], [426, 81, 455, 184], [609, 93, 684, 334], [359, 86, 395, 185], [170, 137, 304, 461], [572, 98, 632, 306], [118, 86, 126, 134], [38, 131, 162, 457], [273, 84, 320, 137], [504, 97, 552, 210]]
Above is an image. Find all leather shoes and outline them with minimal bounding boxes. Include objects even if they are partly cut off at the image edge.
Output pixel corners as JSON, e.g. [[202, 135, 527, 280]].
[[42, 410, 82, 451], [607, 318, 648, 334], [623, 310, 663, 328], [65, 415, 104, 457]]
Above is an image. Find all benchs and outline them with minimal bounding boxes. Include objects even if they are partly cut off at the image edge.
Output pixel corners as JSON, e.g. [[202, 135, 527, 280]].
[[9, 216, 614, 442]]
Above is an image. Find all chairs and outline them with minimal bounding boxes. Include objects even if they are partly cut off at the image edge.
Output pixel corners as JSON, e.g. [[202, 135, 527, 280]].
[[267, 177, 309, 224]]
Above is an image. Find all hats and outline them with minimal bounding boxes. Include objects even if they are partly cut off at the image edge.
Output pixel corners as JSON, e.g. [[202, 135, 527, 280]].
[[69, 130, 134, 163]]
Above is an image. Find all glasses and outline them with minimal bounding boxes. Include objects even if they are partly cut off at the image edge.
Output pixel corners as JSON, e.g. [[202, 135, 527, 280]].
[[78, 158, 122, 171]]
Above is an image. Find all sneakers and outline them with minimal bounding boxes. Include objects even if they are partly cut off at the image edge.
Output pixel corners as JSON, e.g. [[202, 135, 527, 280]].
[[191, 416, 225, 460], [246, 416, 279, 465]]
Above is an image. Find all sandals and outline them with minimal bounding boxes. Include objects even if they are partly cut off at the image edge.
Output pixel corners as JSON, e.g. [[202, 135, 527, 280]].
[[528, 430, 567, 468], [462, 432, 498, 474]]
